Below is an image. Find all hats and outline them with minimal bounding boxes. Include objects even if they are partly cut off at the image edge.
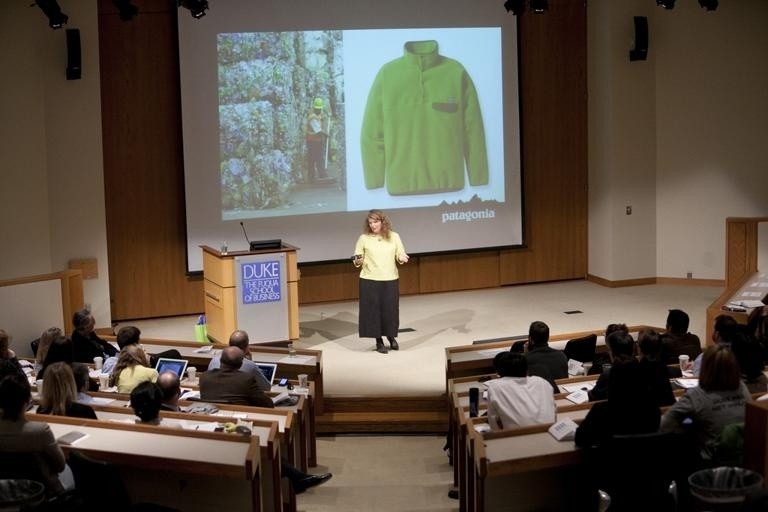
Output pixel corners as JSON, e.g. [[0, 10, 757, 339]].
[[312, 97, 324, 109]]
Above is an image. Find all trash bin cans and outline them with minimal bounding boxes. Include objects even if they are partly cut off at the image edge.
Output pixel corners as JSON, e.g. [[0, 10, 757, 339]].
[[688, 465, 767, 511]]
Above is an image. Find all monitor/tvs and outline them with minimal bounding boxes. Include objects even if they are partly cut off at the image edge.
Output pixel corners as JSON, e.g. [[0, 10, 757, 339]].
[[251, 238, 282, 250]]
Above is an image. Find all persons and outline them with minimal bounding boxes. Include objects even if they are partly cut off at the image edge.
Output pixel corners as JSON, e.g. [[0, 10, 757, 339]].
[[306, 98, 330, 180], [353, 209, 410, 353]]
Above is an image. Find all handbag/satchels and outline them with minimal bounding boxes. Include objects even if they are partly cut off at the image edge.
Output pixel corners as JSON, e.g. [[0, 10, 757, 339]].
[[194, 315, 211, 343]]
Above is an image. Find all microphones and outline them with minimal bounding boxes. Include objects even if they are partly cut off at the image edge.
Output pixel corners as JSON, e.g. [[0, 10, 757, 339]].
[[239, 221, 253, 251]]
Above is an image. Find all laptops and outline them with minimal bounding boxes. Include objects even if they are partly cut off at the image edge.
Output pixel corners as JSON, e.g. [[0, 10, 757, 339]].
[[153, 357, 188, 381], [256, 361, 278, 385]]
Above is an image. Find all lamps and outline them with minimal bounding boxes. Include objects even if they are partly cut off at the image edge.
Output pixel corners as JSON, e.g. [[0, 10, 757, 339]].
[[173, 0, 212, 20], [28, 1, 70, 30], [503, 0, 718, 15]]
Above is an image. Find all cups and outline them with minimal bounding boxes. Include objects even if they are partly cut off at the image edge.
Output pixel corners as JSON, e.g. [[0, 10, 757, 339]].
[[93, 356, 103, 370], [602, 363, 611, 375], [298, 374, 308, 386], [218, 240, 228, 256], [288, 344, 297, 358], [187, 367, 196, 381], [98, 373, 109, 389], [469, 387, 479, 418], [679, 355, 690, 371], [31, 368, 38, 385], [36, 379, 43, 396]]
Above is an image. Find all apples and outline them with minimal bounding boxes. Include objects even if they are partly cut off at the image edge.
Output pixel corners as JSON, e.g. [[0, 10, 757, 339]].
[[224, 421, 236, 432]]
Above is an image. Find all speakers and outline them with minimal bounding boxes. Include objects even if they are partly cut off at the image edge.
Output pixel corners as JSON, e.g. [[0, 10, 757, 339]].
[[631, 15, 650, 61], [65, 28, 82, 80]]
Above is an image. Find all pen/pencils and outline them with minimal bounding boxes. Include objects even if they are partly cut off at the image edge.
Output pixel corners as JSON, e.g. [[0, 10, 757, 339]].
[[195, 426, 201, 431]]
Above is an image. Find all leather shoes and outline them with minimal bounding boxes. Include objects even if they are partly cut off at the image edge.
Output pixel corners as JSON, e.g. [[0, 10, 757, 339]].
[[376, 342, 388, 353], [386, 335, 400, 350]]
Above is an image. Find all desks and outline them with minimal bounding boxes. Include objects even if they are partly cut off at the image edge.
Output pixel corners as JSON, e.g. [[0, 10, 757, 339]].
[[2, 334, 326, 512], [448, 268, 766, 510]]
